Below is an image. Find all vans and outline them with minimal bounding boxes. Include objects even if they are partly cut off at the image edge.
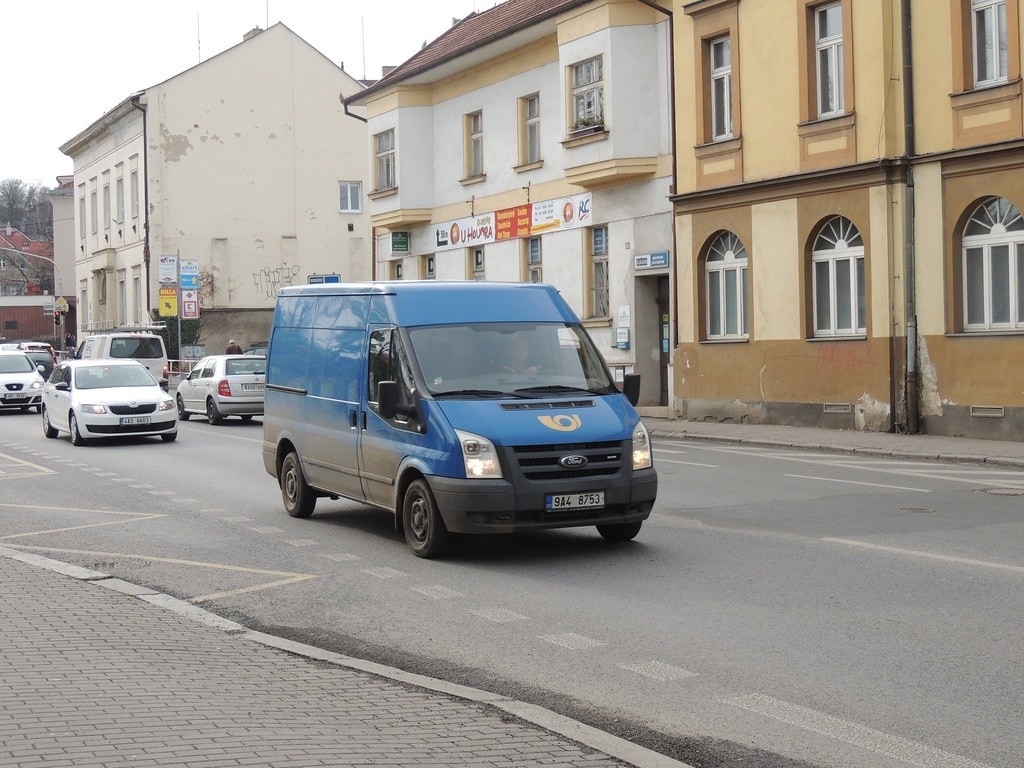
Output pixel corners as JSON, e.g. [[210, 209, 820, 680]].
[[67, 331, 169, 393], [263, 279, 658, 558]]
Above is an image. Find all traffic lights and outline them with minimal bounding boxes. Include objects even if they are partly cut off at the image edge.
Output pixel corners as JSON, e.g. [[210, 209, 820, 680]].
[[61, 310, 65, 315], [54, 310, 62, 326]]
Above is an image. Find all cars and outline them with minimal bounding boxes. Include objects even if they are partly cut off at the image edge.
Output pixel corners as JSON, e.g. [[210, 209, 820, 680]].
[[0, 340, 56, 381], [176, 353, 268, 429], [40, 358, 179, 446], [0, 351, 44, 414]]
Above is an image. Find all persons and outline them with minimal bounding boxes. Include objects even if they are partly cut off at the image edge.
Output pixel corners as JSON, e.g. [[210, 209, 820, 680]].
[[65, 333, 76, 357], [225, 339, 243, 354], [497, 333, 542, 374]]
